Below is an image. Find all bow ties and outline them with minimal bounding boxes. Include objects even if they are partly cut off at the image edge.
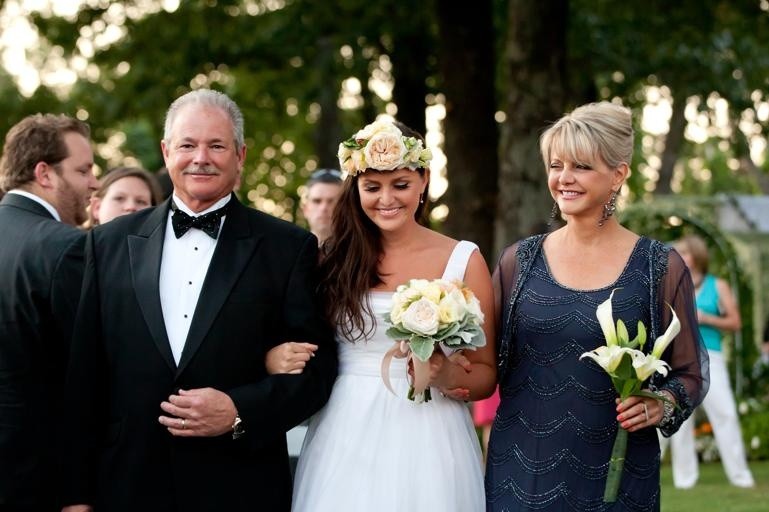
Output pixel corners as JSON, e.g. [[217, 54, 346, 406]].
[[171, 208, 221, 239]]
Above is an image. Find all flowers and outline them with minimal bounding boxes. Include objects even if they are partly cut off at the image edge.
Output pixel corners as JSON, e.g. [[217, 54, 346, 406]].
[[381, 279, 487, 402], [338, 121, 433, 176], [578, 286, 680, 503]]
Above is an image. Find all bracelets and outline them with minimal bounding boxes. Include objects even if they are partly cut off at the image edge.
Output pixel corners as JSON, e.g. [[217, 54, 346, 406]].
[[655, 390, 674, 427]]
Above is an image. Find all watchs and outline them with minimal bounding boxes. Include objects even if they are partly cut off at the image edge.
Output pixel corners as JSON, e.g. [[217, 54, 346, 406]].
[[231, 413, 245, 440]]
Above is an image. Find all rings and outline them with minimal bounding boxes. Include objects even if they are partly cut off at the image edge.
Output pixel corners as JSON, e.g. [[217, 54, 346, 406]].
[[439, 391, 447, 399]]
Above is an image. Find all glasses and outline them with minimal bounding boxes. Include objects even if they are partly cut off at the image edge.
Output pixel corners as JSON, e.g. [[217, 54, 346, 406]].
[[310, 168, 341, 179]]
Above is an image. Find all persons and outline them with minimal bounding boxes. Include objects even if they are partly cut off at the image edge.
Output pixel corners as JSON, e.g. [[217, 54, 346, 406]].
[[0, 113, 99, 512], [265, 116, 502, 512], [52, 87, 338, 511], [428, 102, 706, 511], [299, 167, 343, 255], [658, 235, 756, 491], [83, 167, 163, 229]]
